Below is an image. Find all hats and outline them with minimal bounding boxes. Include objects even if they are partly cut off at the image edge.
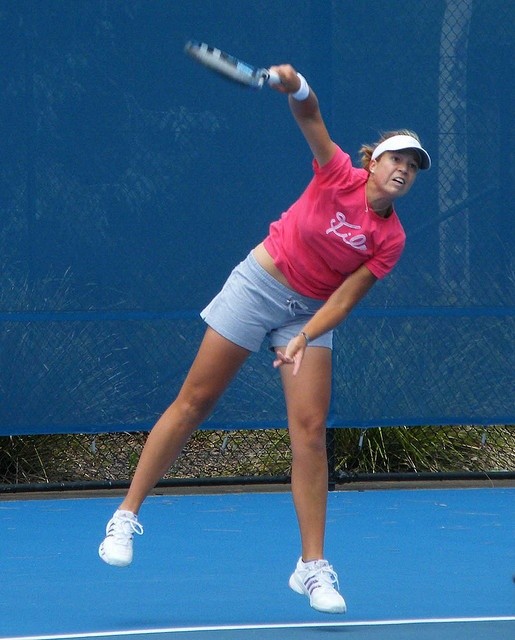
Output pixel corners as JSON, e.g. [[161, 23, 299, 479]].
[[371, 135, 432, 171]]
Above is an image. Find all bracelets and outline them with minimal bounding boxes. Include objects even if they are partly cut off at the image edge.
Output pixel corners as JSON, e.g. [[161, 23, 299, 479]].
[[289, 71, 310, 101], [298, 331, 311, 345]]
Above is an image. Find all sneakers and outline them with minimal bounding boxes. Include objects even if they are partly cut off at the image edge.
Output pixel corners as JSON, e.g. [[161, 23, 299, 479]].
[[289, 557, 348, 614], [98, 508, 144, 568]]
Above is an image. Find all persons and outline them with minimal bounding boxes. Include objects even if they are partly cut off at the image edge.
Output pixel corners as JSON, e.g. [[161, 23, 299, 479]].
[[99, 63, 432, 616]]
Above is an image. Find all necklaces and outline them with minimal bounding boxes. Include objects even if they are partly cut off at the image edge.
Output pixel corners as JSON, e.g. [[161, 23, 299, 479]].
[[361, 179, 393, 214]]
